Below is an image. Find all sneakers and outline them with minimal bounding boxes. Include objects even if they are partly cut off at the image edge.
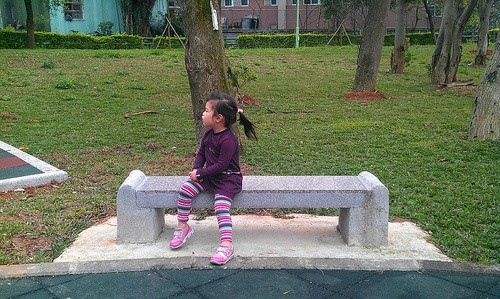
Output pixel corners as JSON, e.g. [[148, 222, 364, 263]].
[[169, 223, 194, 248], [209, 244, 234, 264]]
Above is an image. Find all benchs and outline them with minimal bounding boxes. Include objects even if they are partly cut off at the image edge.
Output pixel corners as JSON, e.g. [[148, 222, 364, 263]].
[[117, 170, 389, 248]]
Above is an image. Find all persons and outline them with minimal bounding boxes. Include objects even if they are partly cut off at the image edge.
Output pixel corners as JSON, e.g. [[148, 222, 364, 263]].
[[169, 93, 260, 265]]
